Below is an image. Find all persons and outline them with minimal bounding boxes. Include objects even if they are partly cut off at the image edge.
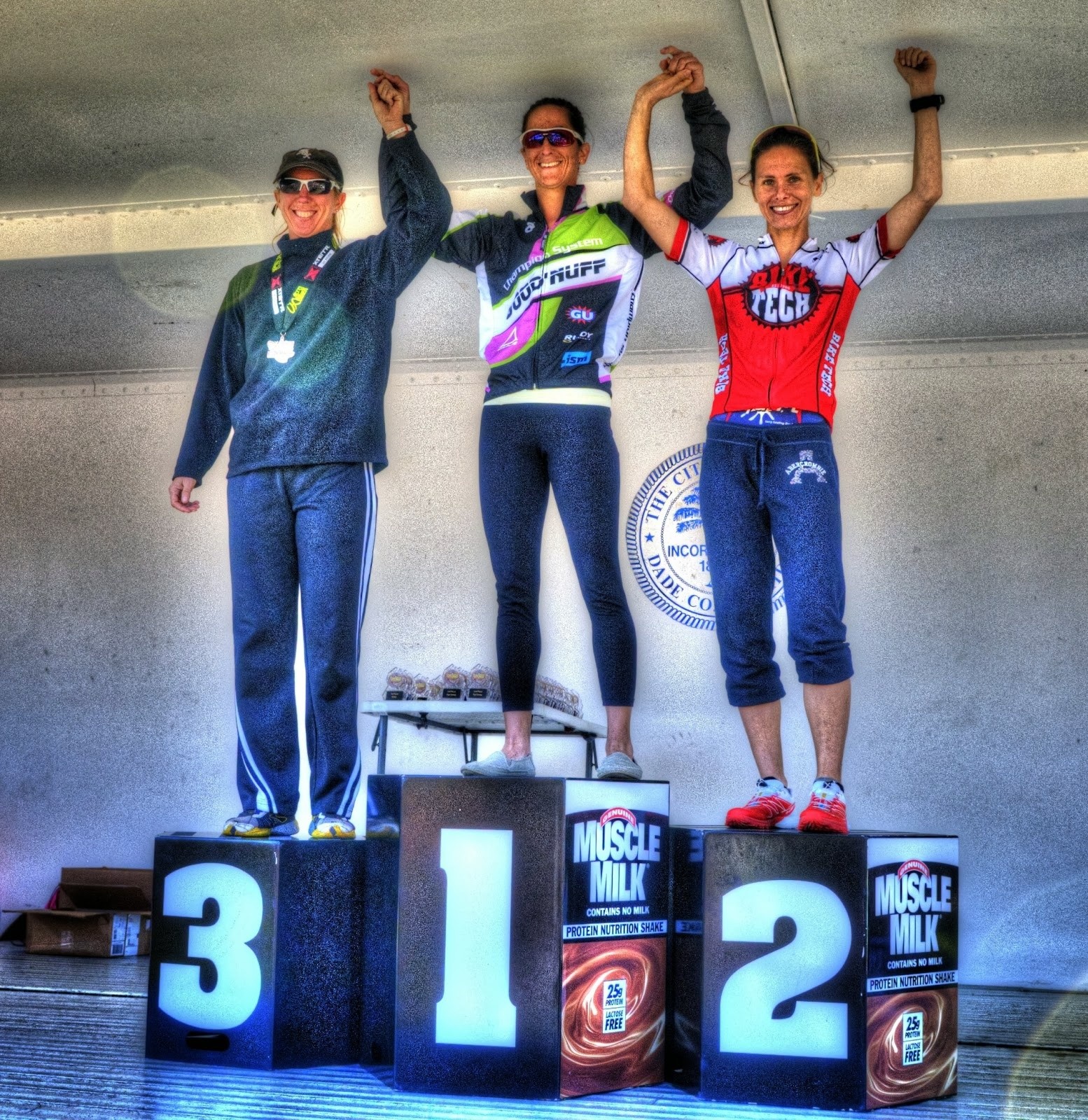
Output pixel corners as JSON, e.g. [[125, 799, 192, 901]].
[[370, 44, 733, 781], [621, 45, 945, 836], [168, 77, 453, 840]]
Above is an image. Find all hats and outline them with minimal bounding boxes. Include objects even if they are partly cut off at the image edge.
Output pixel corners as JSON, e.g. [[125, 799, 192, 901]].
[[750, 125, 821, 174], [271, 148, 343, 193]]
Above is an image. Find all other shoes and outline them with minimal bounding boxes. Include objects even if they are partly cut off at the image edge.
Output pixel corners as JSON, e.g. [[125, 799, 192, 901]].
[[596, 751, 644, 781], [460, 750, 536, 777]]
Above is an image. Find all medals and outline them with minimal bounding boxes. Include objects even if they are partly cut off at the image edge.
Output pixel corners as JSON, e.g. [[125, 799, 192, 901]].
[[265, 338, 296, 364]]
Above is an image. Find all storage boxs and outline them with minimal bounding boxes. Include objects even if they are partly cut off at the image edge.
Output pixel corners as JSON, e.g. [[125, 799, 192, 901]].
[[0, 865, 154, 958]]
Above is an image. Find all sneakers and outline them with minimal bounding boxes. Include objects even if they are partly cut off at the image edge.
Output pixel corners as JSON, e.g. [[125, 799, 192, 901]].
[[307, 812, 355, 839], [367, 813, 399, 840], [724, 778, 801, 829], [797, 780, 849, 834], [222, 807, 300, 838]]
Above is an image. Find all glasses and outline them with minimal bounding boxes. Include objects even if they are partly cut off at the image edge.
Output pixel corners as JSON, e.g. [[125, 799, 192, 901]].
[[277, 177, 340, 195], [518, 127, 585, 148]]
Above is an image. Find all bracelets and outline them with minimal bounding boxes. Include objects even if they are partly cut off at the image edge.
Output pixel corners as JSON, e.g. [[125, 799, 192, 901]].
[[385, 125, 413, 140], [910, 93, 945, 113]]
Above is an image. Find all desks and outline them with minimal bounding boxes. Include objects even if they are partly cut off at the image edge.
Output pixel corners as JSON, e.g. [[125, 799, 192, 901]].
[[362, 698, 613, 778]]
